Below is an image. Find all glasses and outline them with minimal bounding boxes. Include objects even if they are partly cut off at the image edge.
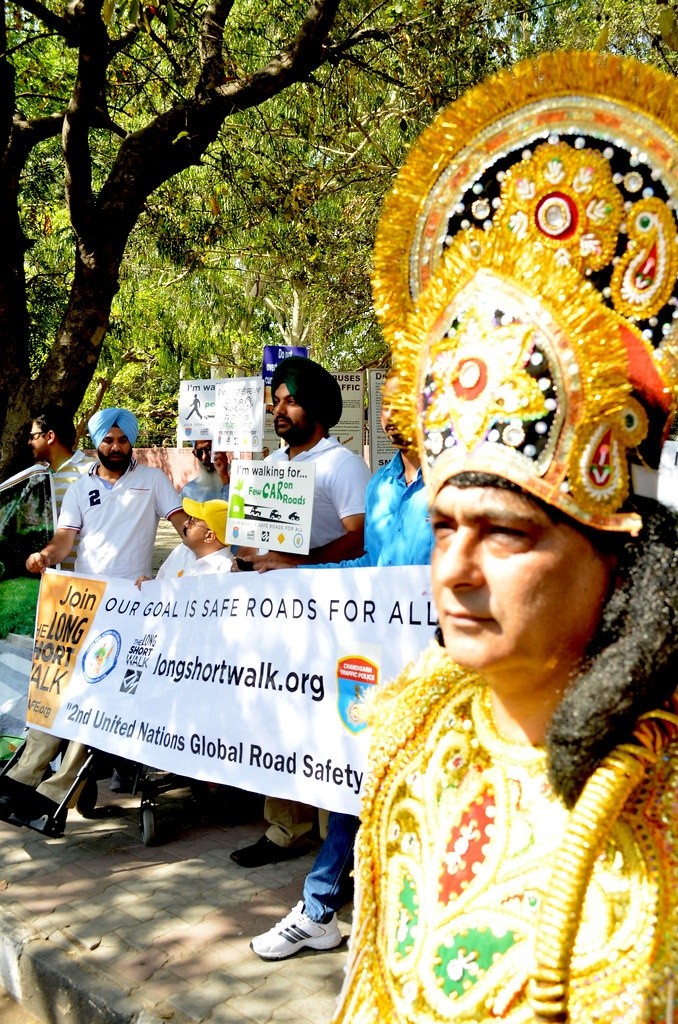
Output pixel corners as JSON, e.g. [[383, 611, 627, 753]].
[[187, 518, 209, 530], [193, 446, 211, 458], [28, 431, 45, 440]]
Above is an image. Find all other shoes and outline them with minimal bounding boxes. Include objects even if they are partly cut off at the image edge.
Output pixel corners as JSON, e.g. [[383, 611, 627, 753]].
[[230, 835, 307, 867], [0, 774, 36, 819], [1, 791, 67, 827]]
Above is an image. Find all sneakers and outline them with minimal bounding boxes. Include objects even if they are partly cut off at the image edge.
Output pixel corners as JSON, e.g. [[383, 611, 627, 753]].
[[249, 900, 342, 961]]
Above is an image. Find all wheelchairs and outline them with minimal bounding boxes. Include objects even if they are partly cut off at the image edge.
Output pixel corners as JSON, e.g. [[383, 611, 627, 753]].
[[0, 737, 265, 848]]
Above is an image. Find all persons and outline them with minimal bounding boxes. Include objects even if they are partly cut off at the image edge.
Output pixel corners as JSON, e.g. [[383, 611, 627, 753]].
[[1, 356, 437, 962], [329, 232, 676, 1024]]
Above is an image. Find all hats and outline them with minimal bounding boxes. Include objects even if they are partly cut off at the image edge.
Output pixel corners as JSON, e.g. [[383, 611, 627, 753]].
[[182, 496, 230, 546], [271, 356, 342, 428], [190, 440, 197, 447], [88, 408, 138, 449]]
